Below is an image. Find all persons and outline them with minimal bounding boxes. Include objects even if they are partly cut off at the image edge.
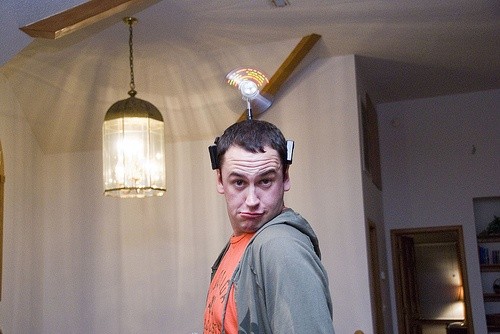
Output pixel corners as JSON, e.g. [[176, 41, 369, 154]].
[[203, 120, 336, 334]]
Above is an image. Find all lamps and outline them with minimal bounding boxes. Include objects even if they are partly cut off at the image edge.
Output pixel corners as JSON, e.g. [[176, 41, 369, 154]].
[[102, 15, 167, 198]]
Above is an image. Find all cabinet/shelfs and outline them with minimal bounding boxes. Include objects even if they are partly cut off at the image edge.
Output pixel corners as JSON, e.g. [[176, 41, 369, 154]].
[[472, 196, 500, 334]]
